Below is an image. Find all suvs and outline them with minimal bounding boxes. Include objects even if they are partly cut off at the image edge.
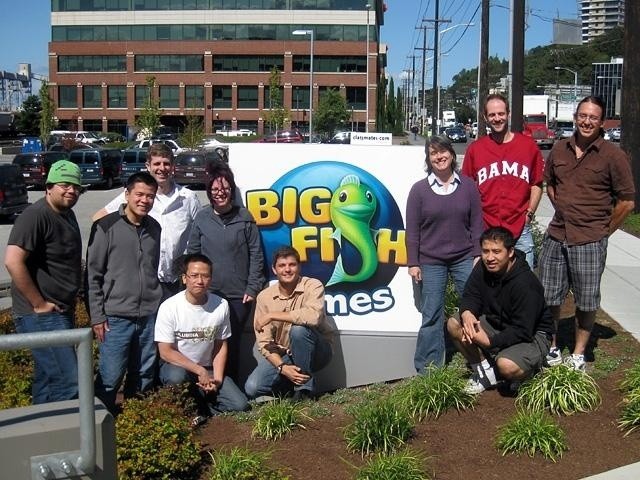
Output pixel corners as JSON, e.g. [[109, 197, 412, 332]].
[[250, 129, 304, 142], [549, 119, 576, 139]]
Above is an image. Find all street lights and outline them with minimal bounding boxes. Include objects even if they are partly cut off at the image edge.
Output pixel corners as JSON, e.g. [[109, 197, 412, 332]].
[[402, 67, 433, 130], [596, 75, 622, 118], [536, 85, 556, 89], [292, 29, 315, 142], [365, 4, 372, 133], [554, 66, 577, 134], [422, 53, 450, 135], [437, 21, 474, 137]]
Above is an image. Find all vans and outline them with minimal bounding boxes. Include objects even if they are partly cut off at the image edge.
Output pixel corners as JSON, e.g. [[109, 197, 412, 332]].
[[119, 147, 150, 188], [68, 148, 123, 189], [318, 131, 351, 144], [0, 162, 32, 218], [48, 130, 229, 158], [12, 152, 69, 189]]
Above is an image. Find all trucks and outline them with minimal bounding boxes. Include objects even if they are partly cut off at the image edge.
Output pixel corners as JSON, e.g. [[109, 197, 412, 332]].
[[215, 129, 256, 136], [20, 136, 45, 152], [521, 94, 554, 150]]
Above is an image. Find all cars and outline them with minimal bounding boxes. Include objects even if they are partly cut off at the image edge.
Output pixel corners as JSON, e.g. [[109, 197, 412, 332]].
[[173, 151, 224, 187], [12, 133, 31, 146], [603, 129, 610, 141], [607, 127, 620, 142], [425, 110, 491, 143]]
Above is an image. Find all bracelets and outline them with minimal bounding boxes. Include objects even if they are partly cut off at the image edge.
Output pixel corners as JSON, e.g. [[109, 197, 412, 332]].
[[524, 210, 533, 219], [276, 363, 288, 374]]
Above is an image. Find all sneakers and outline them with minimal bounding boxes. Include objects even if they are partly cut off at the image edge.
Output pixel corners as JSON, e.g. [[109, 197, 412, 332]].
[[564, 354, 586, 370], [466, 366, 497, 395], [546, 347, 562, 367], [293, 390, 311, 401]]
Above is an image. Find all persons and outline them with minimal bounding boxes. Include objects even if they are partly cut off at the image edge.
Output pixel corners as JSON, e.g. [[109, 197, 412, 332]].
[[459, 94, 546, 274], [83, 172, 162, 416], [185, 168, 266, 388], [536, 95, 637, 372], [90, 141, 203, 303], [404, 134, 484, 374], [4, 160, 83, 406], [445, 222, 556, 396], [152, 253, 251, 418], [243, 245, 334, 403]]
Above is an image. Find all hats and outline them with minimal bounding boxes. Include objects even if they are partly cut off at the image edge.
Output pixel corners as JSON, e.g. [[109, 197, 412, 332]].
[[45, 159, 81, 186]]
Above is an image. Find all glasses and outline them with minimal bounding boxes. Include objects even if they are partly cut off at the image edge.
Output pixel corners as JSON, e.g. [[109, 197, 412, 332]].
[[56, 184, 79, 190], [210, 188, 231, 195]]
